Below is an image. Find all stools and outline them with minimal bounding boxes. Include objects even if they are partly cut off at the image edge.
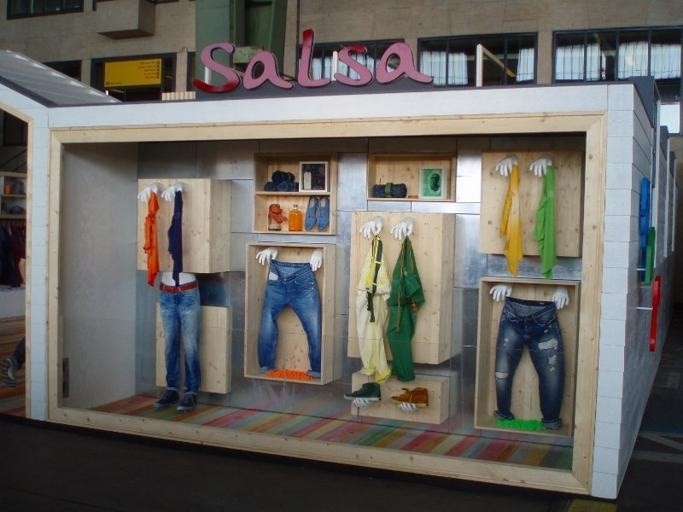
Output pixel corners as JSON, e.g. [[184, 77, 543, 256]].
[[159, 280, 199, 293]]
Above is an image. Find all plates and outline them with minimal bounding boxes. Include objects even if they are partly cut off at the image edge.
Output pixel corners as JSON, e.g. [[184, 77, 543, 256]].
[[243, 241, 336, 387], [478, 147, 585, 259], [365, 150, 458, 203], [346, 210, 456, 366], [474, 274, 582, 440], [350, 370, 450, 426], [135, 177, 232, 275], [0, 169, 27, 220], [250, 149, 339, 236]]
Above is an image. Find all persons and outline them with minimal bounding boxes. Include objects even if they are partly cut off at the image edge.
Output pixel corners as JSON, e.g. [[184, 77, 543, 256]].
[[528, 154, 552, 176], [255, 246, 323, 272], [398, 402, 419, 413], [494, 155, 520, 176], [0, 335, 25, 388], [352, 396, 375, 408], [430, 173, 440, 191], [152, 271, 203, 411], [136, 181, 159, 201], [358, 214, 384, 239], [488, 283, 571, 310], [389, 216, 415, 240], [160, 182, 184, 202]]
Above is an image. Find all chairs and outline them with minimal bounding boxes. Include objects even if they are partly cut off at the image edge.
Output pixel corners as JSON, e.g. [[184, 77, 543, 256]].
[[180, 393, 197, 411], [305, 196, 329, 231], [391, 387, 428, 407], [344, 383, 381, 401], [156, 389, 179, 409], [2, 358, 19, 388]]
